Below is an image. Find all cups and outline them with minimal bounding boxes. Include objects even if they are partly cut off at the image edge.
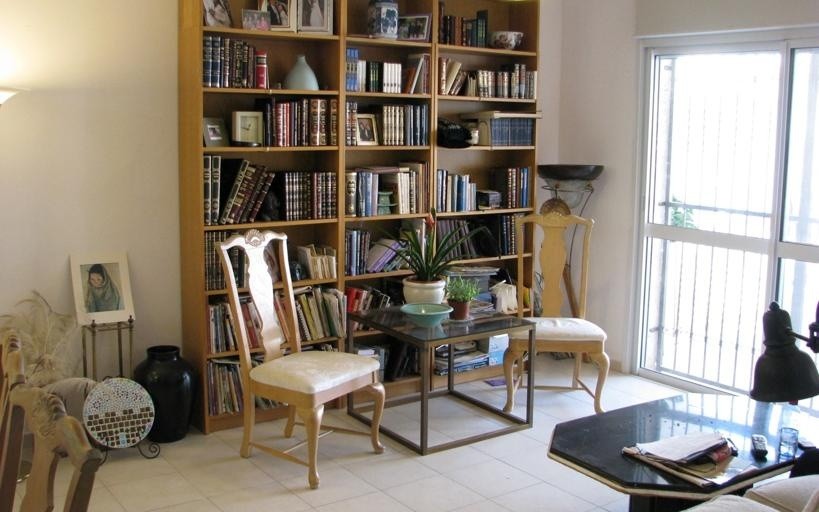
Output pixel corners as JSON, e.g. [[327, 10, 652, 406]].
[[777, 427, 799, 457]]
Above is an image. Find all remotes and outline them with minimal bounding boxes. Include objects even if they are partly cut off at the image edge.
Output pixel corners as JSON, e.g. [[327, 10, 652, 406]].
[[752, 434, 768, 456]]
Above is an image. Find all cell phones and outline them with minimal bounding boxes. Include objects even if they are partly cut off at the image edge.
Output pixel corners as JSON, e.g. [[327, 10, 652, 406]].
[[798, 436, 816, 450]]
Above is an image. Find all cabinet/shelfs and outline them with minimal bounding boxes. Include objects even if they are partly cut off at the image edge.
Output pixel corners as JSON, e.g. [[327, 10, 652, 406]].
[[177, 0, 542, 436], [82, 315, 134, 399]]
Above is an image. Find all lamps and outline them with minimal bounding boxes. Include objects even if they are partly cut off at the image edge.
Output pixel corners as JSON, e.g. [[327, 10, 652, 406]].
[[748, 299, 819, 403]]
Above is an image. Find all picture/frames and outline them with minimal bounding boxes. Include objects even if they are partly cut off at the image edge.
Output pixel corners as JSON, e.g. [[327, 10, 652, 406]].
[[356, 114, 379, 146], [69, 250, 136, 328], [202, 0, 334, 36]]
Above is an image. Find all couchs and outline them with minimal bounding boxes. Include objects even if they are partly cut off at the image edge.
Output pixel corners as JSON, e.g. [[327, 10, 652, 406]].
[[682, 475, 819, 512]]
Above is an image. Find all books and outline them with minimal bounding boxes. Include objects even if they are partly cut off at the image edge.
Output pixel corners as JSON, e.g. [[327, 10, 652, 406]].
[[624, 431, 759, 489], [436, 212, 516, 377], [440, 2, 488, 47], [347, 219, 430, 382], [258, 94, 431, 146], [204, 35, 269, 89], [346, 49, 536, 101], [207, 230, 348, 416], [205, 157, 530, 225], [474, 116, 536, 147]]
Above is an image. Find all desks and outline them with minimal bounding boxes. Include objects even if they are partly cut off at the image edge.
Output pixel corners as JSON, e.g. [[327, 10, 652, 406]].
[[345, 300, 537, 456]]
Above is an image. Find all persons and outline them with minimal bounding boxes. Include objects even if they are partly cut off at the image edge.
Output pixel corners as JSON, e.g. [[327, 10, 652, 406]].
[[397, 20, 425, 38], [86, 263, 122, 312], [244, 1, 324, 30]]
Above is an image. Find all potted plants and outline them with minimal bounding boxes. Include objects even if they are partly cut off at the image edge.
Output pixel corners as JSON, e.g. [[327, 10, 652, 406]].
[[443, 275, 482, 320], [371, 207, 487, 304]]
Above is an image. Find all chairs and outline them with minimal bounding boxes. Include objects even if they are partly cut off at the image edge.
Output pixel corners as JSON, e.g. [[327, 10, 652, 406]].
[[0, 329, 103, 512], [502, 213, 610, 414], [214, 229, 386, 490]]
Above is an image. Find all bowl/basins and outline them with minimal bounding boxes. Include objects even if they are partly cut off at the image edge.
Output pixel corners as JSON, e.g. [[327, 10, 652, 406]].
[[487, 31, 524, 50], [399, 302, 454, 330]]
